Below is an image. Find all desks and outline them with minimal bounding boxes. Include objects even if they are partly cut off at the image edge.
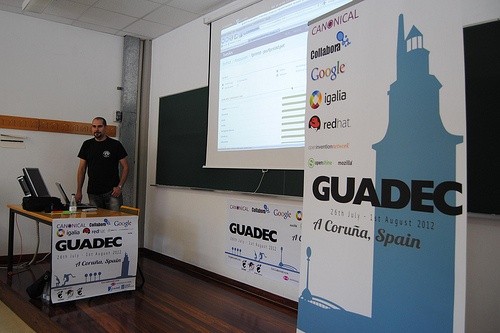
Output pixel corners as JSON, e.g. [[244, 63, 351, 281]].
[[7, 204, 136, 292]]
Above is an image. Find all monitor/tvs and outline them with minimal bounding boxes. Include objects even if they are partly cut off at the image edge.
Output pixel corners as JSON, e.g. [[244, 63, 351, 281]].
[[22, 167, 51, 197]]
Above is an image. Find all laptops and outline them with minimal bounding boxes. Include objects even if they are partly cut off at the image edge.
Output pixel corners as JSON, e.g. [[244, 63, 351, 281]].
[[56, 182, 97, 209]]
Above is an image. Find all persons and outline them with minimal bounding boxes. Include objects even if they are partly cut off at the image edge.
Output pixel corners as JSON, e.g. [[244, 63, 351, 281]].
[[75, 116, 129, 210]]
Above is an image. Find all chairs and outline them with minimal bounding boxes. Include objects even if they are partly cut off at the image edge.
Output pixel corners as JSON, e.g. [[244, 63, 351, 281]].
[[120, 205, 144, 280]]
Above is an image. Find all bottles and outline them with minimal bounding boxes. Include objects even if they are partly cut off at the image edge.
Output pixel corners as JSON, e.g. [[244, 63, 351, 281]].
[[69, 194, 77, 211]]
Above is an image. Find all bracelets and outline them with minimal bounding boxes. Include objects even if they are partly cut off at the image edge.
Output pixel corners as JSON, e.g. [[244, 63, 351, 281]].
[[118, 185, 123, 190]]
